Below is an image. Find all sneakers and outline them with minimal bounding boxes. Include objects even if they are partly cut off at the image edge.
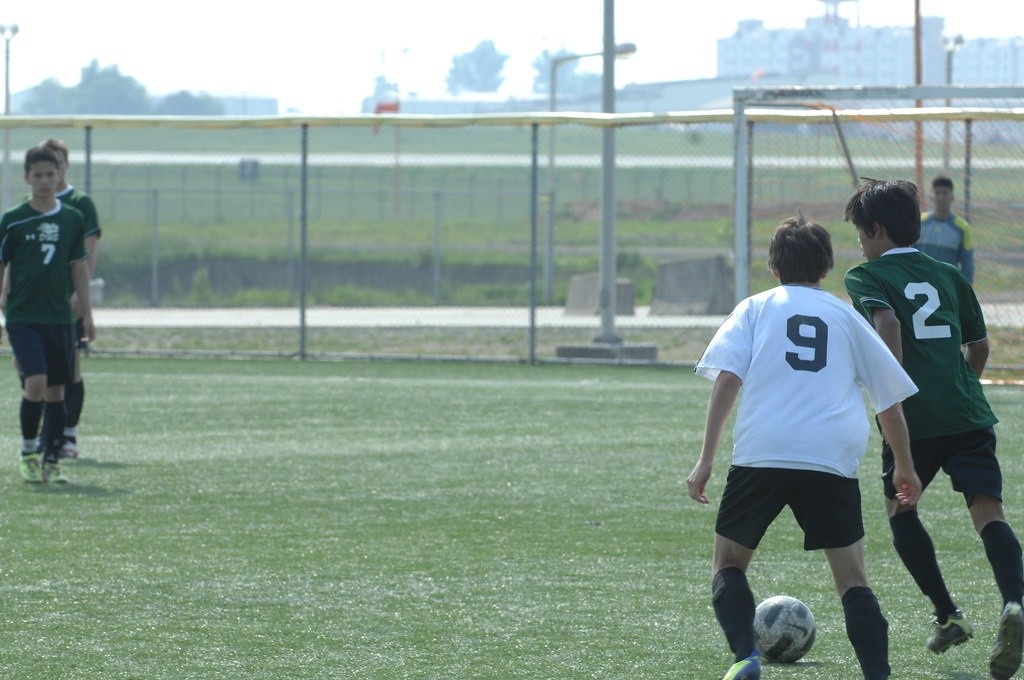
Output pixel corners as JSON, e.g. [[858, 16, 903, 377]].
[[718, 650, 762, 680], [989, 602, 1024, 680], [58, 434, 78, 465], [928, 609, 973, 655], [19, 449, 43, 482], [42, 459, 66, 485]]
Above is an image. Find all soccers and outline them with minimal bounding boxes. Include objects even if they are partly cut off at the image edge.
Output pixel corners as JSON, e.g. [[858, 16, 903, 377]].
[[751, 595, 817, 663]]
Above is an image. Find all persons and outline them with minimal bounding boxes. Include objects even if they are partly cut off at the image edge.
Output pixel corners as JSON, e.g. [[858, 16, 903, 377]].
[[844, 177, 1024, 680], [686, 216, 922, 680], [916, 174, 974, 283], [0, 140, 101, 466], [0, 146, 97, 483]]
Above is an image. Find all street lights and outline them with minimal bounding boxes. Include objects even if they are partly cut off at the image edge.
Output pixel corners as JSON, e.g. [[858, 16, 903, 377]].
[[0, 23, 20, 213], [541, 42, 638, 307], [939, 31, 966, 173]]
